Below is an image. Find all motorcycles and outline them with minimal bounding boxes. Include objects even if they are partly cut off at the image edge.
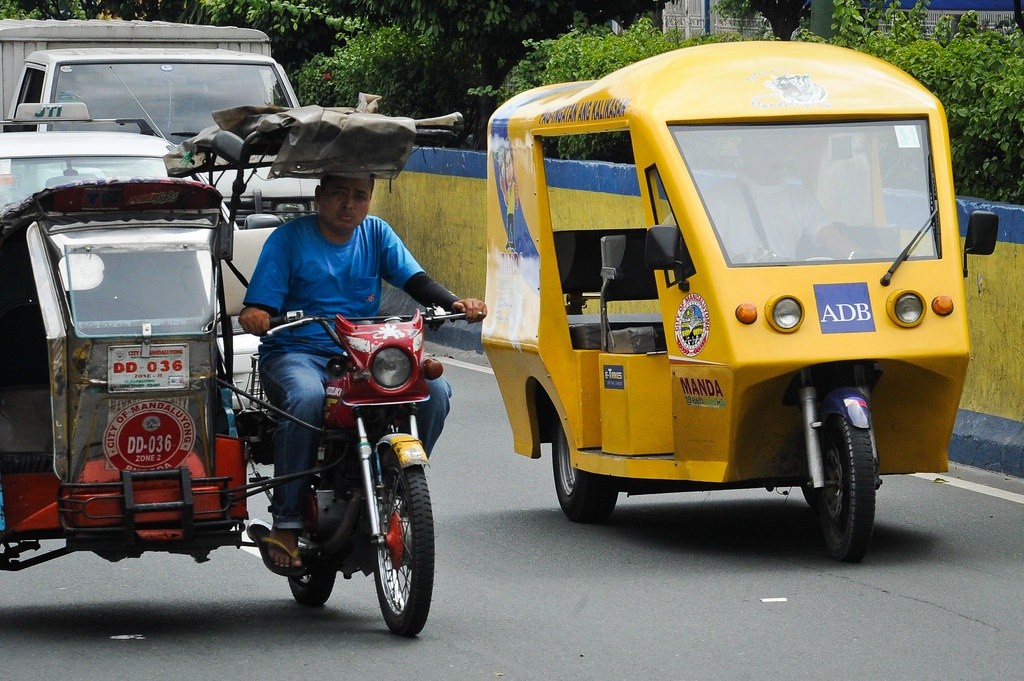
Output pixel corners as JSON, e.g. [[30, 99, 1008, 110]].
[[482, 41, 999, 565], [250, 308, 485, 639]]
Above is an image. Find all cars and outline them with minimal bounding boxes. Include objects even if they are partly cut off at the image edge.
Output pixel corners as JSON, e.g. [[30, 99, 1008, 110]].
[[1, 103, 290, 467]]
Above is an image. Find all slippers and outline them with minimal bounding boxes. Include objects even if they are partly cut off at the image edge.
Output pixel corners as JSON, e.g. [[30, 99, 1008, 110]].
[[248, 524, 308, 577]]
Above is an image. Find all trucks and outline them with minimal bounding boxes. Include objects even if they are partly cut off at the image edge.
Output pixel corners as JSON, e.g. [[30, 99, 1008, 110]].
[[0, 17, 325, 227]]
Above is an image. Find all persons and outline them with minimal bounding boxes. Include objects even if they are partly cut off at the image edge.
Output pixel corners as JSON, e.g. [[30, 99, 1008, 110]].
[[662, 132, 889, 263], [238, 171, 488, 571]]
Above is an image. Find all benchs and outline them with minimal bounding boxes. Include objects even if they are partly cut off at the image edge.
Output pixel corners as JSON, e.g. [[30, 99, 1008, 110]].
[[555, 227, 669, 352]]
